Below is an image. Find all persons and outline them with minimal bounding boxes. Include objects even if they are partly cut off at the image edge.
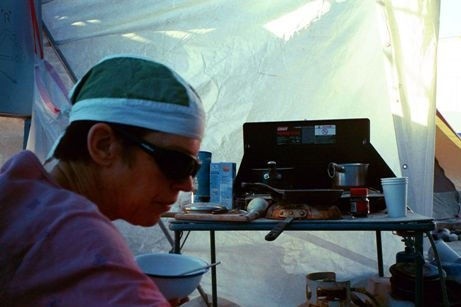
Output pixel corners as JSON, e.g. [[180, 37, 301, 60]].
[[0, 57, 206, 307]]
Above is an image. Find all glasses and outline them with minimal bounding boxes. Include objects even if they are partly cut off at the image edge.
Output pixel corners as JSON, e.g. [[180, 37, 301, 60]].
[[111, 125, 202, 182]]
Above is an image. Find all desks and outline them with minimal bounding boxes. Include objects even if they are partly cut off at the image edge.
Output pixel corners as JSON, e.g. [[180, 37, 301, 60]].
[[168, 211, 448, 307]]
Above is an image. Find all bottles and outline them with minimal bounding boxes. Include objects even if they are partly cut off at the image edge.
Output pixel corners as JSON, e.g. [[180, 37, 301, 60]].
[[350, 187, 370, 218]]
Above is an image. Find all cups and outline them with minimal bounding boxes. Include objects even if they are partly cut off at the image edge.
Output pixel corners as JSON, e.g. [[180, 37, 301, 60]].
[[381, 176, 408, 217], [428, 239, 461, 262]]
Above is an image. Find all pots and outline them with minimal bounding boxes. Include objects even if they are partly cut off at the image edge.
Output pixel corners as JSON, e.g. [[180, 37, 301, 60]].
[[328, 163, 370, 187], [265, 199, 342, 241], [252, 161, 294, 189]]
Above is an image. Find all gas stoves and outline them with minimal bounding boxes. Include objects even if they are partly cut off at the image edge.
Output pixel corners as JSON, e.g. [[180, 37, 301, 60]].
[[234, 187, 386, 215]]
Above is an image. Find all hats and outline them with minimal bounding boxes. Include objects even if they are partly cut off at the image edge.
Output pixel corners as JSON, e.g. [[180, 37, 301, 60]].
[[68, 57, 203, 141]]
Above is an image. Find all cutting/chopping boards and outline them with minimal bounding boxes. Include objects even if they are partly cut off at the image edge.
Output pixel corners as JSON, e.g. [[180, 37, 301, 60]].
[[174, 208, 251, 221]]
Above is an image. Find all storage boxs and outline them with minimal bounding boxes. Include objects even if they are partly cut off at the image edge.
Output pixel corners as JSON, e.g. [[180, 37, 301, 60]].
[[210, 162, 237, 210]]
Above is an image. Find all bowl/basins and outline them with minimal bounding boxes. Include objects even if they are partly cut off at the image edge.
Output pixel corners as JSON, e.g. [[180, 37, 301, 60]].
[[134, 251, 210, 299]]
